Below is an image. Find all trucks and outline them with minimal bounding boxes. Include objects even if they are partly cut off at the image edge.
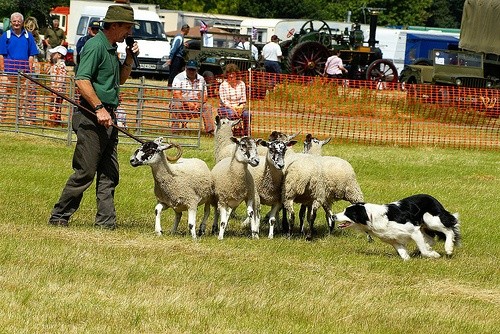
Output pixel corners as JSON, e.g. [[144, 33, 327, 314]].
[[50, 6, 461, 79]]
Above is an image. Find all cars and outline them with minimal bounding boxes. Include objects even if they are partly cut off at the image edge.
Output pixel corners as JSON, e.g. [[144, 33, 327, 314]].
[[167, 30, 264, 99], [403, 49, 500, 107]]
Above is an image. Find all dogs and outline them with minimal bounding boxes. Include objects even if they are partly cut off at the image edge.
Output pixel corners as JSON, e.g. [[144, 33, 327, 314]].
[[329, 192, 463, 263]]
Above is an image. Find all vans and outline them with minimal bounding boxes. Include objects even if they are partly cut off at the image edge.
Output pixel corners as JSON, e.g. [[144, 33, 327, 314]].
[[67, 0, 171, 81]]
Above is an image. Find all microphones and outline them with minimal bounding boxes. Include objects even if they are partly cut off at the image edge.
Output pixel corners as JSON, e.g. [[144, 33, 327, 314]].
[[126, 36, 140, 67]]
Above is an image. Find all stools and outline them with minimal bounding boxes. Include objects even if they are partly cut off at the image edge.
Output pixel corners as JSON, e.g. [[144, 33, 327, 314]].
[[229, 116, 246, 136], [171, 112, 208, 134]]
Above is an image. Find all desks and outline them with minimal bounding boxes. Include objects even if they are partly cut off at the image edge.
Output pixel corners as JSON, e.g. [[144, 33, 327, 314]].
[[199, 63, 223, 76]]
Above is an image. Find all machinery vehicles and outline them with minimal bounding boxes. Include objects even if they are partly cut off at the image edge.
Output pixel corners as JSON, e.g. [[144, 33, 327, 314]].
[[278, 2, 398, 91]]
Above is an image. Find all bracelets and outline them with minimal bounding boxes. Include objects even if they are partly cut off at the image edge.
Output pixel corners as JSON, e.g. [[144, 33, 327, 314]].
[[93, 103, 105, 113]]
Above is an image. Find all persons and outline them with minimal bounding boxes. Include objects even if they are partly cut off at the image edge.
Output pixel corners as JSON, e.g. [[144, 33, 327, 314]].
[[0, 11, 68, 128], [230, 35, 259, 62], [47, 5, 140, 234], [261, 35, 284, 98], [75, 19, 102, 75], [216, 62, 253, 137], [321, 49, 348, 88], [167, 23, 190, 93], [170, 60, 209, 133]]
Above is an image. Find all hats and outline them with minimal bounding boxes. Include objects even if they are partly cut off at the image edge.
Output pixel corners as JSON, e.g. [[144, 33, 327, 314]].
[[49, 46, 67, 56], [271, 35, 277, 40], [98, 5, 140, 25], [185, 59, 198, 70], [89, 21, 101, 29]]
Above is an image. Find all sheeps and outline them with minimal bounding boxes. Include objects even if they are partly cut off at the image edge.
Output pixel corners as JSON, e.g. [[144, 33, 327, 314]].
[[128, 134, 213, 240], [196, 113, 375, 243]]
